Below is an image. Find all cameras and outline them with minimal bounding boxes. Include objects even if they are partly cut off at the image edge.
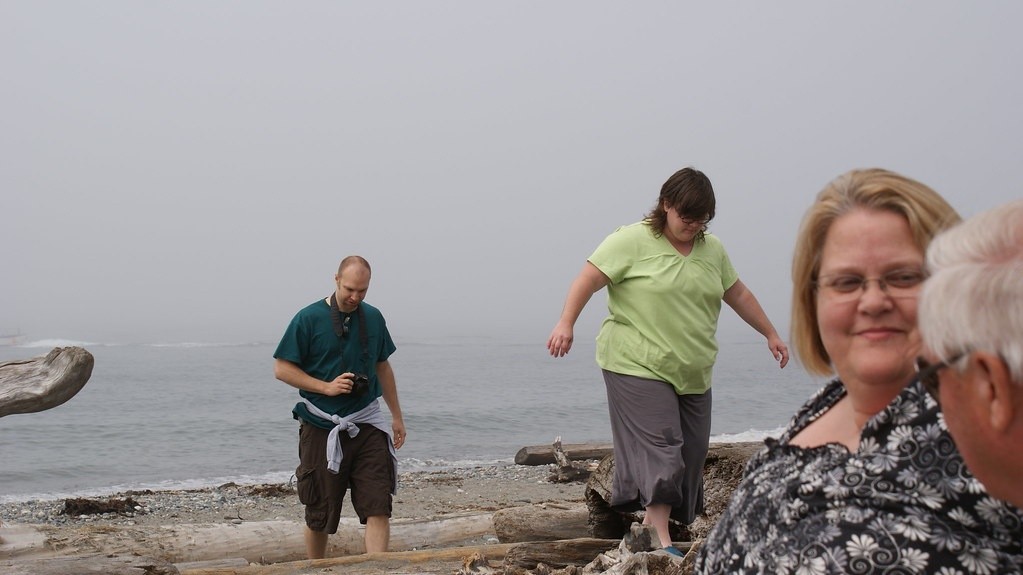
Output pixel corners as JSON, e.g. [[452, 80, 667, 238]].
[[344, 374, 370, 400]]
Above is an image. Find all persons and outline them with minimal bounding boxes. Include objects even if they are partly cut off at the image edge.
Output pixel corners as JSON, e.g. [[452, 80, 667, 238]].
[[272, 256, 407, 559], [546, 167, 790, 558], [694, 169, 1023, 575]]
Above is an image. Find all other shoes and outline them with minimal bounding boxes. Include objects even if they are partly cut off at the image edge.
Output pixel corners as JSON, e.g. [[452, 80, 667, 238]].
[[663, 545, 684, 557]]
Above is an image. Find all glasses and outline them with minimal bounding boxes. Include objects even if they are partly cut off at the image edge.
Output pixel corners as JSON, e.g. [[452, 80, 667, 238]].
[[809, 270, 929, 304], [342, 316, 351, 334], [913, 347, 969, 406], [673, 206, 711, 225]]
